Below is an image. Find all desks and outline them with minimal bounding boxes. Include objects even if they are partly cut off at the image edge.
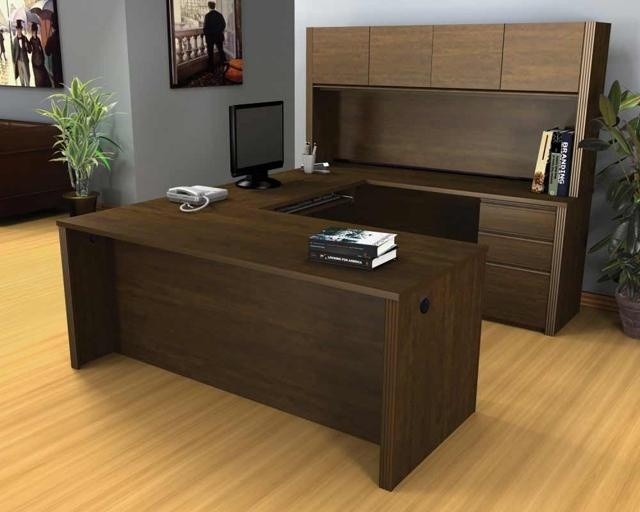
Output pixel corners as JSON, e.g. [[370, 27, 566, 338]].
[[62, 171, 478, 496]]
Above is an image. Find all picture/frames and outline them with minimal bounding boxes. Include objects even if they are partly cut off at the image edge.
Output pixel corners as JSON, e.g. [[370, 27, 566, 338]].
[[167, 0, 242, 89], [0, 0, 65, 88]]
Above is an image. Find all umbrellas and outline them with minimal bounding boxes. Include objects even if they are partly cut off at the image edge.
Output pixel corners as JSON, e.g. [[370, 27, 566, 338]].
[[28, 0, 54, 20], [7, 6, 42, 27], [0, 10, 7, 26]]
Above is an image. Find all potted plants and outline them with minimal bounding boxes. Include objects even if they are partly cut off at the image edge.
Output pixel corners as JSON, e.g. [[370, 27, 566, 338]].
[[37, 76, 130, 217], [577, 81, 640, 339]]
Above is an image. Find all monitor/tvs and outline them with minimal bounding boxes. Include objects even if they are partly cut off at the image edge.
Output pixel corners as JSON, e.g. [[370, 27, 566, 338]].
[[228, 99, 285, 189]]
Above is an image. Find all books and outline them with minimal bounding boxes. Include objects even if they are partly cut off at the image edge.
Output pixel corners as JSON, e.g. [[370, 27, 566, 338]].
[[308, 225, 398, 256], [530, 127, 575, 197], [308, 247, 400, 269]]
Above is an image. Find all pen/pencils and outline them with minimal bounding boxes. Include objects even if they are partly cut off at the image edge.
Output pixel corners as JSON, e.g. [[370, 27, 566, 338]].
[[302, 141, 319, 155]]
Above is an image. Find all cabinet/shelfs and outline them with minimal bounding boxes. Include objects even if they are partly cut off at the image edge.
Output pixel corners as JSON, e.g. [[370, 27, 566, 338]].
[[313, 24, 368, 89], [370, 24, 432, 88], [503, 24, 585, 89], [476, 199, 554, 336], [433, 22, 502, 93]]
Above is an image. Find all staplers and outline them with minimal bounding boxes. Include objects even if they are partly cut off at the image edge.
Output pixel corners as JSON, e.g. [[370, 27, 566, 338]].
[[300, 161, 330, 174]]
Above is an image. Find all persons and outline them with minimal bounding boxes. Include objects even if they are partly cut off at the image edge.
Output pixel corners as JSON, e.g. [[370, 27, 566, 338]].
[[44, 14, 63, 88], [27, 23, 52, 87], [11, 20, 31, 87], [0, 30, 7, 60], [203, 1, 226, 71]]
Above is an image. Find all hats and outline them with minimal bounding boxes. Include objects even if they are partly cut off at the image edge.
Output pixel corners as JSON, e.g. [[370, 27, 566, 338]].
[[32, 24, 37, 30], [16, 20, 23, 28]]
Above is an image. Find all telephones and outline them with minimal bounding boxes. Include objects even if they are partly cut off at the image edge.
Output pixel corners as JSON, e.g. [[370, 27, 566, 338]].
[[167, 185, 228, 206]]
